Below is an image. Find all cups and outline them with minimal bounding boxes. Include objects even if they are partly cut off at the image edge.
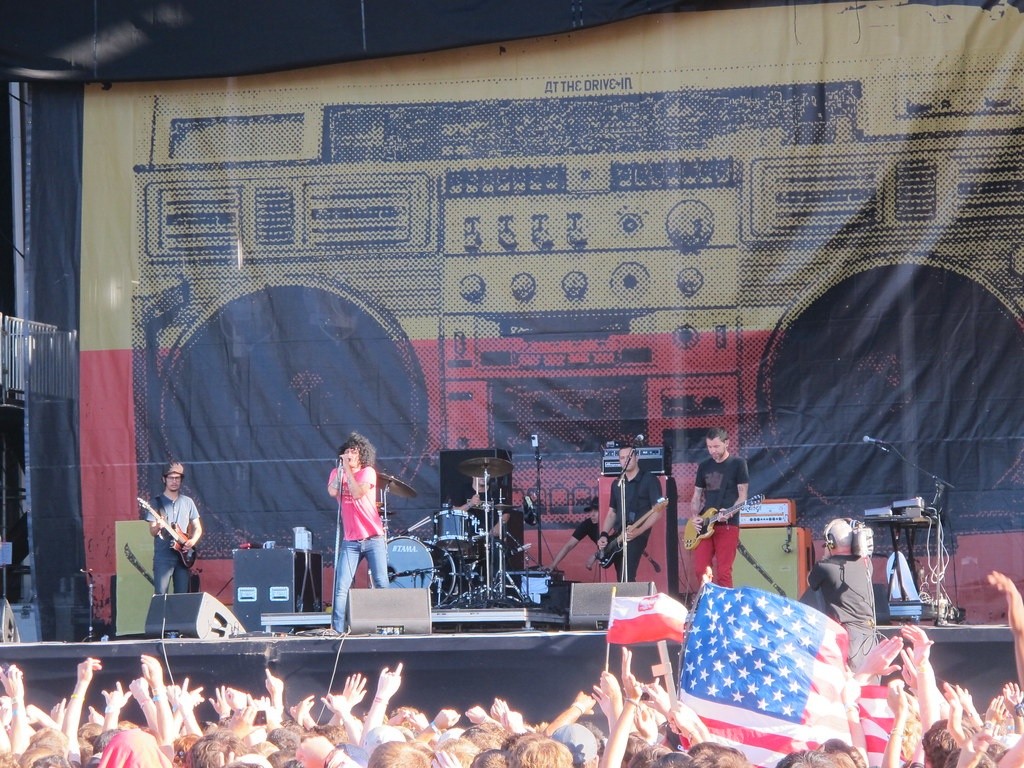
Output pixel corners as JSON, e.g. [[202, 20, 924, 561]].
[[264, 541, 276, 548]]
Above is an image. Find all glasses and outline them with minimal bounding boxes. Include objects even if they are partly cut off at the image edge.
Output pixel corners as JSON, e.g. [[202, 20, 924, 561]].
[[165, 476, 181, 481], [344, 449, 359, 455]]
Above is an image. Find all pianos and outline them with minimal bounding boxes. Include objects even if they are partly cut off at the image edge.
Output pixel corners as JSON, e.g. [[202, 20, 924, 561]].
[[862, 496, 947, 601]]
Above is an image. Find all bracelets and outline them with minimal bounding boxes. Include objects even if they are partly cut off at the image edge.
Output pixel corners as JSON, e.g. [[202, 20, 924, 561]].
[[889, 729, 904, 739], [11, 695, 24, 704], [431, 722, 438, 732], [480, 714, 486, 722], [104, 707, 120, 713], [984, 721, 992, 729], [968, 711, 978, 717], [467, 499, 471, 508], [374, 696, 389, 705], [601, 530, 608, 538], [625, 697, 638, 706], [152, 696, 167, 701], [571, 702, 586, 715], [152, 687, 166, 694], [13, 709, 26, 716], [687, 730, 697, 742], [70, 695, 84, 701], [730, 513, 734, 517], [140, 698, 151, 708]]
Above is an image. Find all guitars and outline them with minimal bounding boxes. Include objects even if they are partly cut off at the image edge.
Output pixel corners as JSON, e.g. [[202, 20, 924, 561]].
[[135, 496, 196, 569], [598, 495, 668, 569], [683, 493, 766, 551]]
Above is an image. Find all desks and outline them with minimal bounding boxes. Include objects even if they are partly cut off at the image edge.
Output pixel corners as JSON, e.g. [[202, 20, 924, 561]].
[[865, 516, 937, 603]]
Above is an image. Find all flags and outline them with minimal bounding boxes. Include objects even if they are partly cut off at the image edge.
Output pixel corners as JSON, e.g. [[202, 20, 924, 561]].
[[606, 592, 689, 644], [860, 686, 911, 768], [678, 579, 851, 768]]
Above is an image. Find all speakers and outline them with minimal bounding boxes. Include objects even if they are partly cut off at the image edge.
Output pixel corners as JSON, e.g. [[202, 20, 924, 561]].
[[145, 592, 246, 641], [569, 582, 657, 630], [346, 589, 431, 635], [733, 528, 813, 603], [0, 598, 21, 643]]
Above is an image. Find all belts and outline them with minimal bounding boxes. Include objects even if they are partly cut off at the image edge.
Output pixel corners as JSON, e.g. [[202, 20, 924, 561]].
[[349, 535, 381, 543]]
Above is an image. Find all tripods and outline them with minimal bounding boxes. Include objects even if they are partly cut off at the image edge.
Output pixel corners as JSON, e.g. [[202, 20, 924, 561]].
[[447, 467, 529, 608], [79, 569, 98, 642]]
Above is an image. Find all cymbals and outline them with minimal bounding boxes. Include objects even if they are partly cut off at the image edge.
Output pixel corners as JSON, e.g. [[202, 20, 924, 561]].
[[376, 502, 396, 515], [490, 503, 521, 510], [457, 456, 515, 478], [376, 471, 419, 498]]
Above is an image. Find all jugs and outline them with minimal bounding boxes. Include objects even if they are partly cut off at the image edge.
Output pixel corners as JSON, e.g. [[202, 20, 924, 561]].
[[292, 526, 308, 550]]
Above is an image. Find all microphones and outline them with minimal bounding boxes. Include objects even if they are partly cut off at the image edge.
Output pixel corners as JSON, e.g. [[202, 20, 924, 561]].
[[336, 458, 343, 462], [635, 435, 644, 441], [388, 572, 394, 580], [785, 549, 792, 553], [863, 436, 883, 444]]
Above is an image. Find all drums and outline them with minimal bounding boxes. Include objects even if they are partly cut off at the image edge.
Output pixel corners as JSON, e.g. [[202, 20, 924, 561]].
[[434, 508, 474, 551], [455, 517, 479, 560], [367, 535, 457, 608]]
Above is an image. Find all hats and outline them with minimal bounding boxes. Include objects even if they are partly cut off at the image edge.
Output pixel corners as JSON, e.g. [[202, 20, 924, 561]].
[[551, 724, 597, 764], [163, 461, 184, 475], [362, 725, 404, 759], [335, 744, 369, 768], [435, 728, 465, 751], [393, 726, 415, 739]]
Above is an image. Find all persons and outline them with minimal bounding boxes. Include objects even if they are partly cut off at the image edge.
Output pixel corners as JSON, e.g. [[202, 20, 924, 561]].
[[0, 571, 1024, 768], [145, 428, 877, 681]]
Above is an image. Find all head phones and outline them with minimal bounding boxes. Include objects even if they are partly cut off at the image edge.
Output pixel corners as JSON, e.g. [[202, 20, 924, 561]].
[[821, 519, 843, 550]]
